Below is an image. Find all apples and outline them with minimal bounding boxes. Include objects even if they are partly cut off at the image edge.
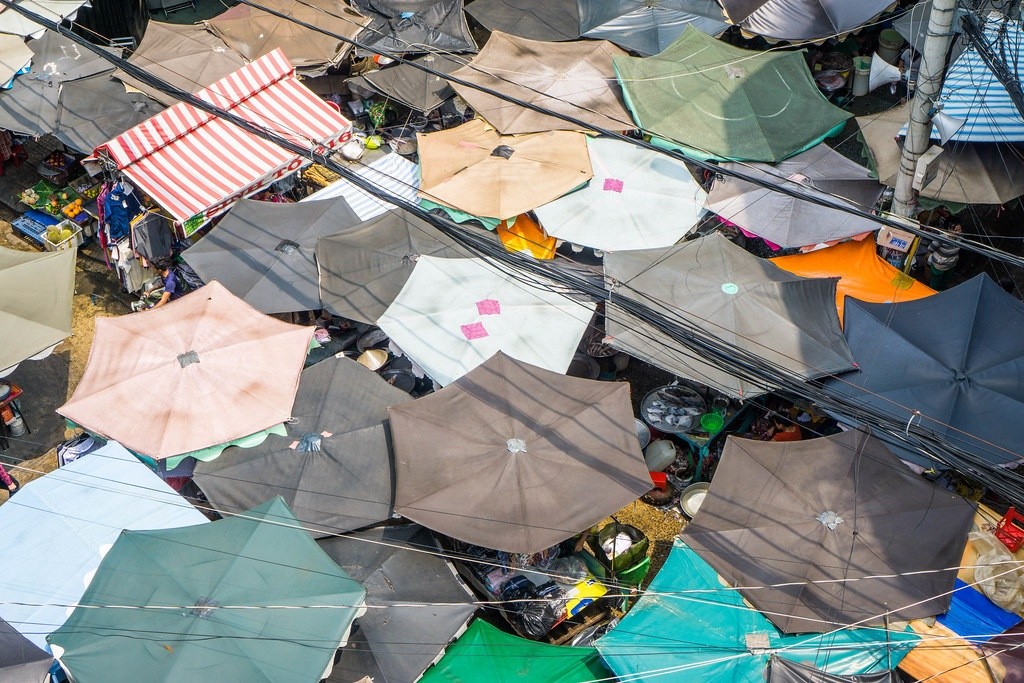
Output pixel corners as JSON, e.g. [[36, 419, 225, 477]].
[[51, 192, 69, 207], [50, 153, 65, 167]]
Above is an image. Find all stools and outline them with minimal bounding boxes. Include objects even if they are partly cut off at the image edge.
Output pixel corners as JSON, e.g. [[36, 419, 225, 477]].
[[996, 508, 1024, 552]]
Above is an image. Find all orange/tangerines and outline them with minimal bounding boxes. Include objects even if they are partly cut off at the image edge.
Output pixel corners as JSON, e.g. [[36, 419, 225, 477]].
[[63, 199, 83, 218], [83, 190, 99, 198]]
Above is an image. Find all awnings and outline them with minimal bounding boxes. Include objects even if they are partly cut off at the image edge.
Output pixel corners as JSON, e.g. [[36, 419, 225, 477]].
[[79, 47, 354, 239]]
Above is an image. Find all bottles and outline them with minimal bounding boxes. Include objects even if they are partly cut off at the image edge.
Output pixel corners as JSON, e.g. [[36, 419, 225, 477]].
[[644, 438, 677, 472]]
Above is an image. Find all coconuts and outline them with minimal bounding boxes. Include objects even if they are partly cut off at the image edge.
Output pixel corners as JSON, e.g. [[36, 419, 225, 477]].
[[46, 225, 72, 244]]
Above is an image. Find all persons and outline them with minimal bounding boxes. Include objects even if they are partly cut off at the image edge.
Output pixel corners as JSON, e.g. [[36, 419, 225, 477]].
[[0, 464, 20, 498], [143, 262, 184, 310], [766, 411, 802, 442], [0, 132, 13, 170], [259, 190, 295, 203], [890, 47, 921, 106], [368, 101, 400, 133], [574, 525, 599, 558], [923, 223, 963, 289]]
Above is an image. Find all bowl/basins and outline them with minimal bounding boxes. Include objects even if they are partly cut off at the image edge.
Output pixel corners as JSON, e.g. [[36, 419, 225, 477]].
[[680, 482, 711, 518], [0, 384, 12, 402]]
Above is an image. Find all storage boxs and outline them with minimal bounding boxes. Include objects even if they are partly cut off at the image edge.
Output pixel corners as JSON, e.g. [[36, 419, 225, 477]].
[[40, 148, 102, 253]]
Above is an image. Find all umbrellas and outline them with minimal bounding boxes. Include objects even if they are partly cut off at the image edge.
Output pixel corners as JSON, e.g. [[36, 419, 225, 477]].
[[0, 0, 1024, 683]]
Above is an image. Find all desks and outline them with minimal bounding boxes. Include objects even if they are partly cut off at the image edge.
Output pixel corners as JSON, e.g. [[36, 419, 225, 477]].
[[0, 383, 31, 448], [640, 380, 765, 482]]
